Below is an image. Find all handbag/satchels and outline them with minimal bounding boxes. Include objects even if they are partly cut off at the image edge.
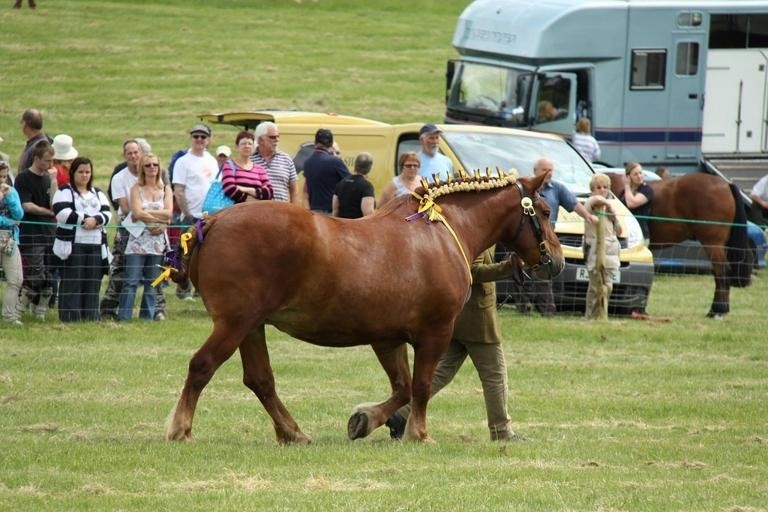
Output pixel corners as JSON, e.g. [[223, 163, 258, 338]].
[[201, 181, 235, 215], [0, 230, 17, 256]]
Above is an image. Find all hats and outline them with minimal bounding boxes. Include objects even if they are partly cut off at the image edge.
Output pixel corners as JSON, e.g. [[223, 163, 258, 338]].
[[419, 124, 443, 136], [189, 124, 211, 137], [51, 134, 78, 161], [216, 145, 231, 158]]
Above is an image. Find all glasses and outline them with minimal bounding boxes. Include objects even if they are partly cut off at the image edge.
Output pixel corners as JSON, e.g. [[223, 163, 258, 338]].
[[404, 164, 417, 168], [194, 135, 207, 139]]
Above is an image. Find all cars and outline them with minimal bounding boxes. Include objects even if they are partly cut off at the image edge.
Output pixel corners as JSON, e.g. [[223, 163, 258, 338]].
[[593, 167, 768, 287]]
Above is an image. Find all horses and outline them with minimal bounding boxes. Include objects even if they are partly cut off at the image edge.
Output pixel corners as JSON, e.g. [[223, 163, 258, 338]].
[[169, 168, 566, 447], [599, 171, 752, 320]]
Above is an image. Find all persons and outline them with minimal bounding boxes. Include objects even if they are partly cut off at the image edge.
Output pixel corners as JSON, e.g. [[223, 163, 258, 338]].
[[385, 242, 526, 443], [510, 158, 599, 319], [582, 175, 622, 321], [1, 109, 300, 326], [306, 122, 452, 220], [747, 174, 768, 229], [538, 100, 557, 121], [619, 160, 655, 247], [572, 117, 600, 163], [655, 167, 669, 178]]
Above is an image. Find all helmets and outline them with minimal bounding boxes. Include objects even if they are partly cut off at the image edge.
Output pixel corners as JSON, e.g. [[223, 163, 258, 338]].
[[356, 153, 372, 169]]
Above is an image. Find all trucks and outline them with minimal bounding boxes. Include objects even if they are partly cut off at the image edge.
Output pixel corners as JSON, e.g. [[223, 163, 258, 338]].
[[195, 109, 655, 317], [442, 0, 768, 227]]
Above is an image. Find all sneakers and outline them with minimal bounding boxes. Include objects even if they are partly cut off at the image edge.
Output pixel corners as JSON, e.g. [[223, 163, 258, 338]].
[[386, 412, 406, 441]]
